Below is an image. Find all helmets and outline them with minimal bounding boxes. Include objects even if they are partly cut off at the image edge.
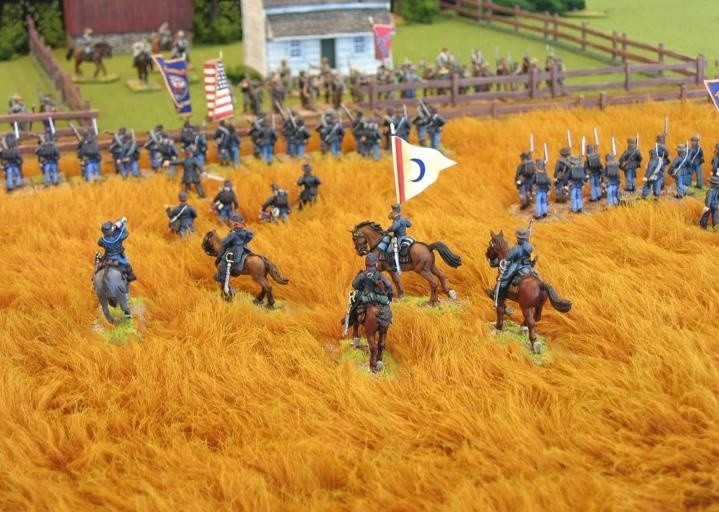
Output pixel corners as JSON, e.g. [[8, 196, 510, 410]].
[[303, 165, 312, 172], [272, 184, 280, 190], [231, 215, 243, 223], [390, 203, 401, 211], [515, 228, 531, 239], [184, 146, 193, 152], [101, 222, 111, 233], [517, 134, 718, 185], [365, 252, 378, 267], [178, 192, 186, 201], [224, 180, 231, 188]]
